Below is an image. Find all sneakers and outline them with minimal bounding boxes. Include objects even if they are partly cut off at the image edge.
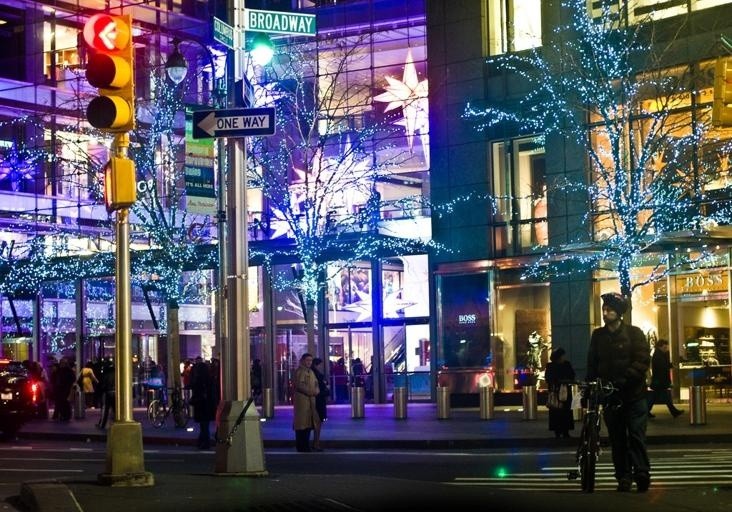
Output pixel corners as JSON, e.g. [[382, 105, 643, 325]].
[[617, 476, 648, 492]]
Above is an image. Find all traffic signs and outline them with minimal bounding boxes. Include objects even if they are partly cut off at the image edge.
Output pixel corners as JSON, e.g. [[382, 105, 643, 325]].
[[243, 7, 317, 38], [213, 17, 233, 50], [192, 106, 277, 141]]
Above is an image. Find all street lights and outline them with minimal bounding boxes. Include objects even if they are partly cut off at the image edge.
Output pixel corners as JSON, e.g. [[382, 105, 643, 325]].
[[167, 31, 229, 404]]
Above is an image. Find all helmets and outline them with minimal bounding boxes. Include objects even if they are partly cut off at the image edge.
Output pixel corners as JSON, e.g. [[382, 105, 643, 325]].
[[601, 293, 626, 313]]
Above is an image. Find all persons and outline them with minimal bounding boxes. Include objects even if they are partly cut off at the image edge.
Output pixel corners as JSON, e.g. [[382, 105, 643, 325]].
[[328, 356, 375, 400], [311, 358, 330, 450], [250, 358, 299, 404], [292, 353, 320, 452], [4, 354, 118, 429], [139, 363, 169, 406], [586, 293, 652, 494], [546, 347, 575, 440], [180, 356, 221, 448], [647, 340, 687, 418]]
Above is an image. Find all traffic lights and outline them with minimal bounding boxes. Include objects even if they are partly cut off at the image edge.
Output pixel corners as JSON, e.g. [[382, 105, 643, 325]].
[[100, 154, 138, 210], [82, 14, 137, 133], [711, 56, 732, 127]]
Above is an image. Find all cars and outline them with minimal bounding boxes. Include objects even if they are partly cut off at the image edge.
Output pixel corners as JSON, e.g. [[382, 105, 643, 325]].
[[1, 367, 41, 441]]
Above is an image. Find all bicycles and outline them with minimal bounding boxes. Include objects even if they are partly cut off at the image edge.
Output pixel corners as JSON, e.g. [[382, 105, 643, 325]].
[[148, 385, 192, 428], [562, 378, 625, 492]]
